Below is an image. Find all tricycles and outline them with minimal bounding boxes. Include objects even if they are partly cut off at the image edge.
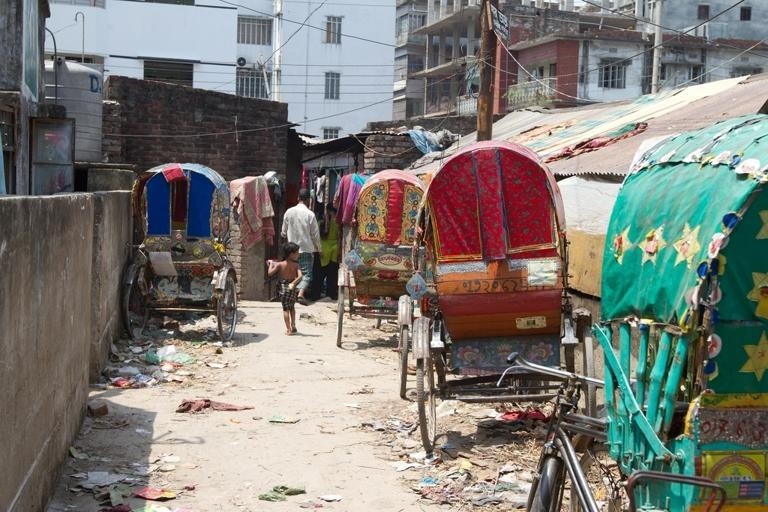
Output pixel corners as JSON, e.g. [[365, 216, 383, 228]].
[[120, 162, 238, 342]]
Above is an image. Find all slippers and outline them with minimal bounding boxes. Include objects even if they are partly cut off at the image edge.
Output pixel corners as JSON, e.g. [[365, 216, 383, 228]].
[[297, 297, 307, 305]]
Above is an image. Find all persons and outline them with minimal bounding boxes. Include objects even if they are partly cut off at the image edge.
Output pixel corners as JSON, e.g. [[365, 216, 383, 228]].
[[266, 242, 303, 336], [281, 188, 324, 306], [311, 202, 341, 301]]
